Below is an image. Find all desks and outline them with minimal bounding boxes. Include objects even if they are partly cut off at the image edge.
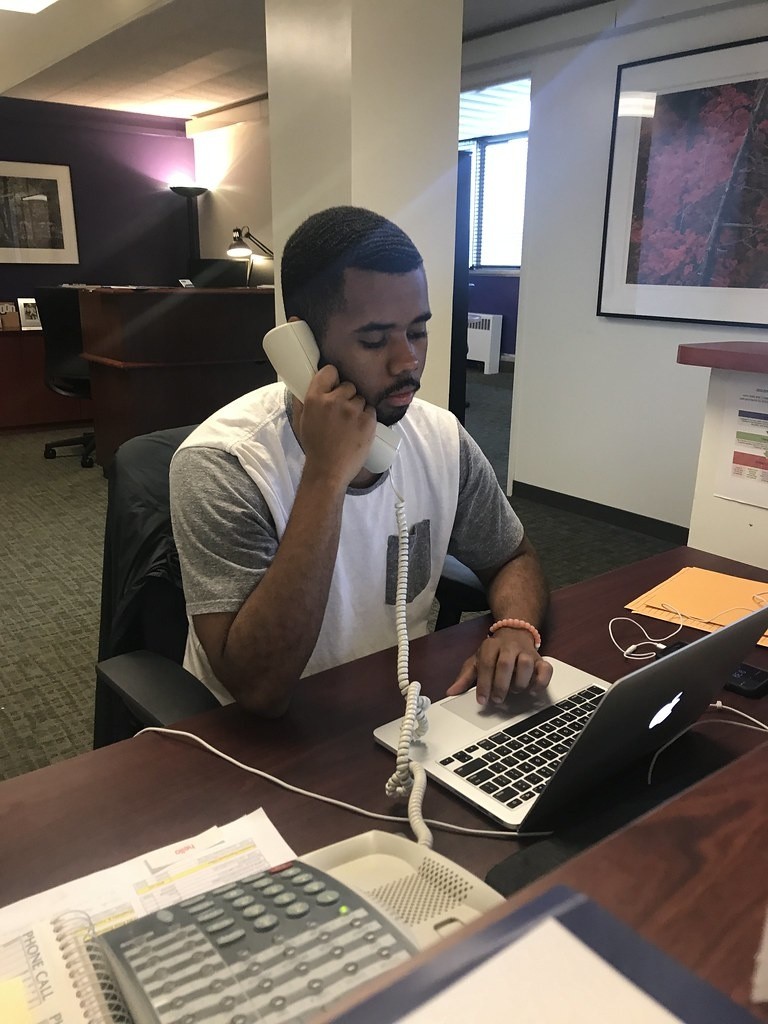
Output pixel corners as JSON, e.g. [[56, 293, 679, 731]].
[[74, 283, 274, 458], [678, 341, 768, 572], [2, 545, 768, 1022]]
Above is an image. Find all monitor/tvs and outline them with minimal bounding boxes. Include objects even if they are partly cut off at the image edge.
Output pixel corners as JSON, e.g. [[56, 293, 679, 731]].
[[189, 258, 250, 288]]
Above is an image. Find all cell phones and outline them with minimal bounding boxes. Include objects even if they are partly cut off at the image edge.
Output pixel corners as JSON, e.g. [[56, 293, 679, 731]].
[[655, 641, 768, 700]]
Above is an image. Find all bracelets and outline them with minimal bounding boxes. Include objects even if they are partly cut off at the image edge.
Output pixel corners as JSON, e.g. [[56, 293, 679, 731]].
[[488, 620, 542, 651]]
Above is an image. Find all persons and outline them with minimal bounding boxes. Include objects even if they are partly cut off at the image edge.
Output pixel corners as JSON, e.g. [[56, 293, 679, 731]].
[[169, 204, 553, 709]]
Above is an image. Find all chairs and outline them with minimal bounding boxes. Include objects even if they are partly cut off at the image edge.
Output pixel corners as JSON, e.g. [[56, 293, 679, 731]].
[[35, 287, 95, 468], [96, 425, 488, 728]]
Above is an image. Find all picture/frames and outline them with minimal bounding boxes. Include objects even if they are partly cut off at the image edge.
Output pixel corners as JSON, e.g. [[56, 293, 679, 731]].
[[1, 161, 79, 265], [17, 298, 42, 332], [596, 35, 768, 327]]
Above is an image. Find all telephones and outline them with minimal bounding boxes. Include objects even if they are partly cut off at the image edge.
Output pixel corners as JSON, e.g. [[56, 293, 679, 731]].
[[262, 319, 403, 475]]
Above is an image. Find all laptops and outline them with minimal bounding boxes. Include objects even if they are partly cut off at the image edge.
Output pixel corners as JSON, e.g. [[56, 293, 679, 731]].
[[374, 607, 768, 835]]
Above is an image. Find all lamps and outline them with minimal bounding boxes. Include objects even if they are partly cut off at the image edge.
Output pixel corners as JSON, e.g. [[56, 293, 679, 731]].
[[226, 225, 273, 270], [170, 186, 249, 286]]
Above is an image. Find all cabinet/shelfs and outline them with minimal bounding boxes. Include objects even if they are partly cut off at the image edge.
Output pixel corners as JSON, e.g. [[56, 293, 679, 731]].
[[0, 330, 90, 432]]
